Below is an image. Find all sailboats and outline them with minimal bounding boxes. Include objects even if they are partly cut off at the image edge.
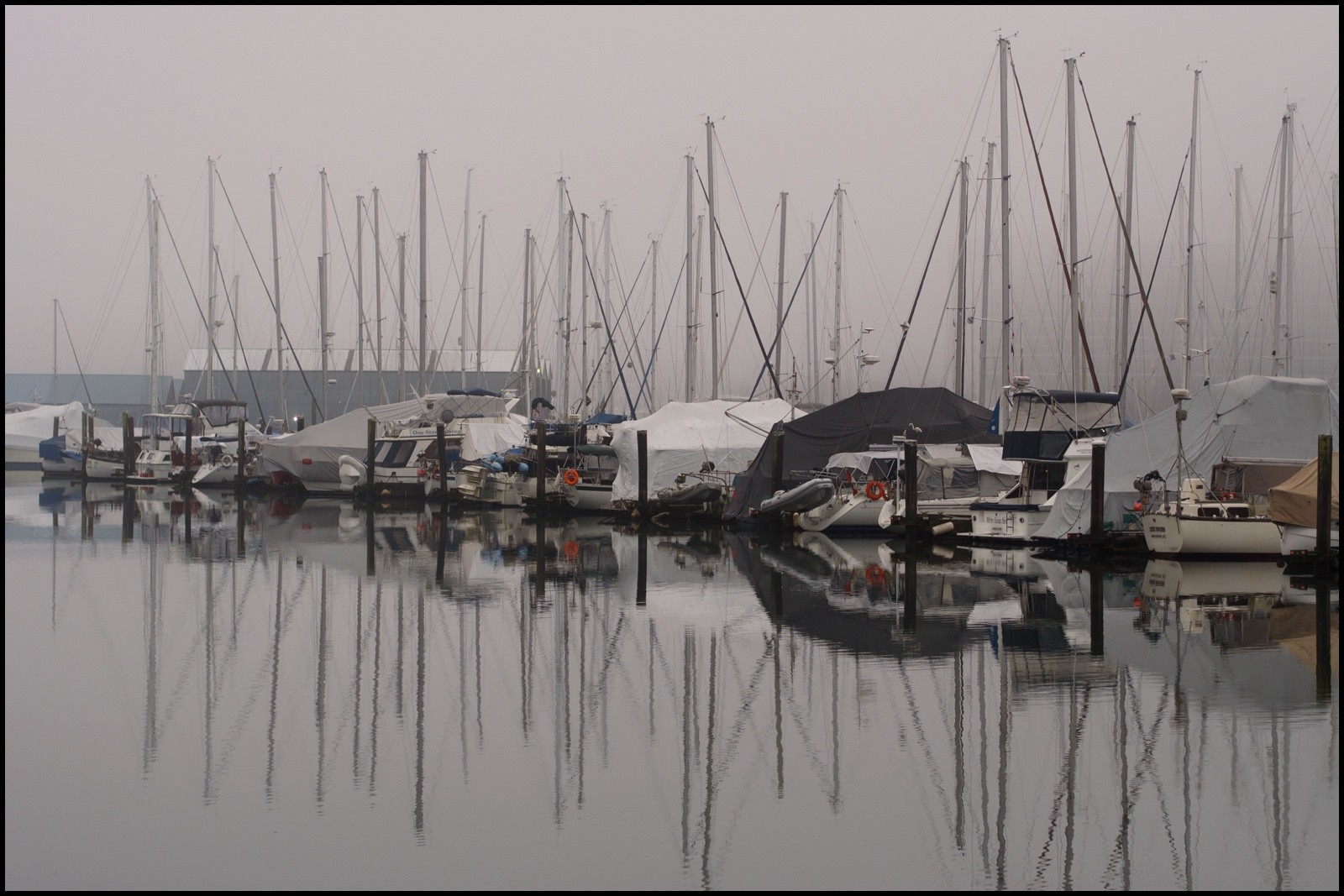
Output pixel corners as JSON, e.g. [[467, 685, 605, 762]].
[[5, 33, 1339, 554]]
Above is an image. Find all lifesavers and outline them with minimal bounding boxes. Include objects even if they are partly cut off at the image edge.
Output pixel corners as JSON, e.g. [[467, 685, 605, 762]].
[[433, 467, 441, 479], [866, 481, 883, 500], [882, 570, 887, 589], [565, 470, 579, 485], [183, 502, 195, 512], [881, 482, 889, 501], [565, 541, 578, 557], [221, 455, 233, 467], [866, 565, 882, 583], [183, 453, 196, 466], [221, 508, 233, 517]]
